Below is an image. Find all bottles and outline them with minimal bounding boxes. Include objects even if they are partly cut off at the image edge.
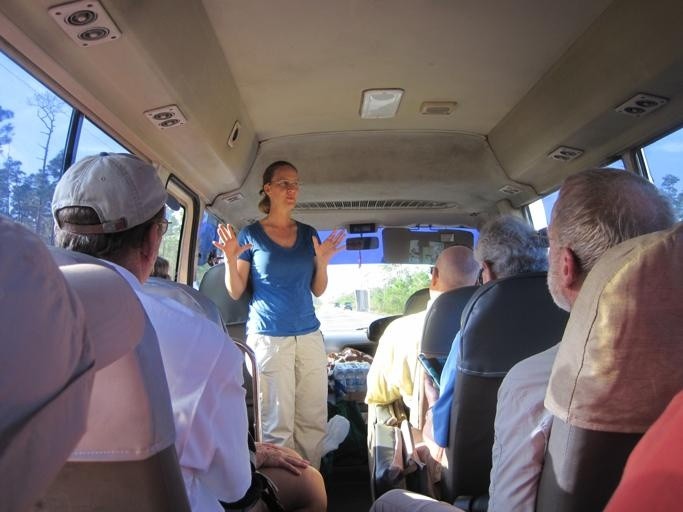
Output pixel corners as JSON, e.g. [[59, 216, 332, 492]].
[[332, 361, 372, 394]]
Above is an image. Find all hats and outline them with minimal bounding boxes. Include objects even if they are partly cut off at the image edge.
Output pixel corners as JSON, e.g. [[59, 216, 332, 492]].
[[51, 153, 181, 235]]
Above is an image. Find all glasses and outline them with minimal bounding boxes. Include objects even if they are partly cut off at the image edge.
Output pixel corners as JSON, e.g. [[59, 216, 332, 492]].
[[159, 216, 172, 236], [271, 179, 304, 190]]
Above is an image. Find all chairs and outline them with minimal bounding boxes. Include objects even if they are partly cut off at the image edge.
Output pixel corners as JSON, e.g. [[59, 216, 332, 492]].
[[393, 286, 477, 432], [435, 270, 569, 504], [35, 243, 193, 510], [536, 220, 683, 512], [145, 275, 225, 330], [202, 264, 250, 344]]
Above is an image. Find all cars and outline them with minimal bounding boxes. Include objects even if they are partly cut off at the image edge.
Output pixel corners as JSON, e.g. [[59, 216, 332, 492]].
[[343, 302, 352, 310]]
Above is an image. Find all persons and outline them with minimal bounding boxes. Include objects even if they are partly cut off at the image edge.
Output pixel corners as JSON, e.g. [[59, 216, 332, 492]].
[[150, 257, 327, 512], [212, 160, 347, 470], [365, 169, 683, 512], [51, 153, 252, 512]]
